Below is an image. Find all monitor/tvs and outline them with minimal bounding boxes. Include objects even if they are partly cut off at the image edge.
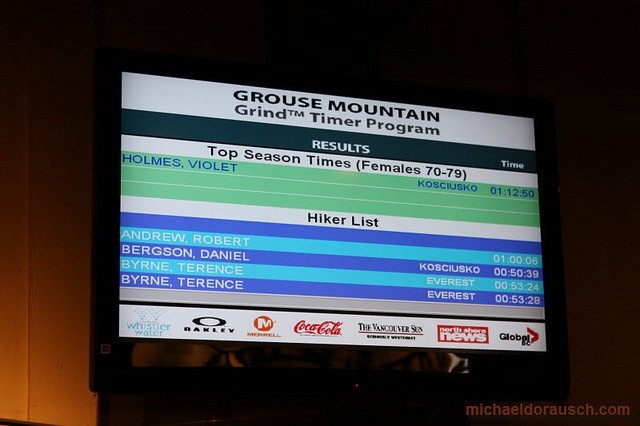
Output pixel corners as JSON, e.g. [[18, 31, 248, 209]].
[[88, 42, 570, 406]]
